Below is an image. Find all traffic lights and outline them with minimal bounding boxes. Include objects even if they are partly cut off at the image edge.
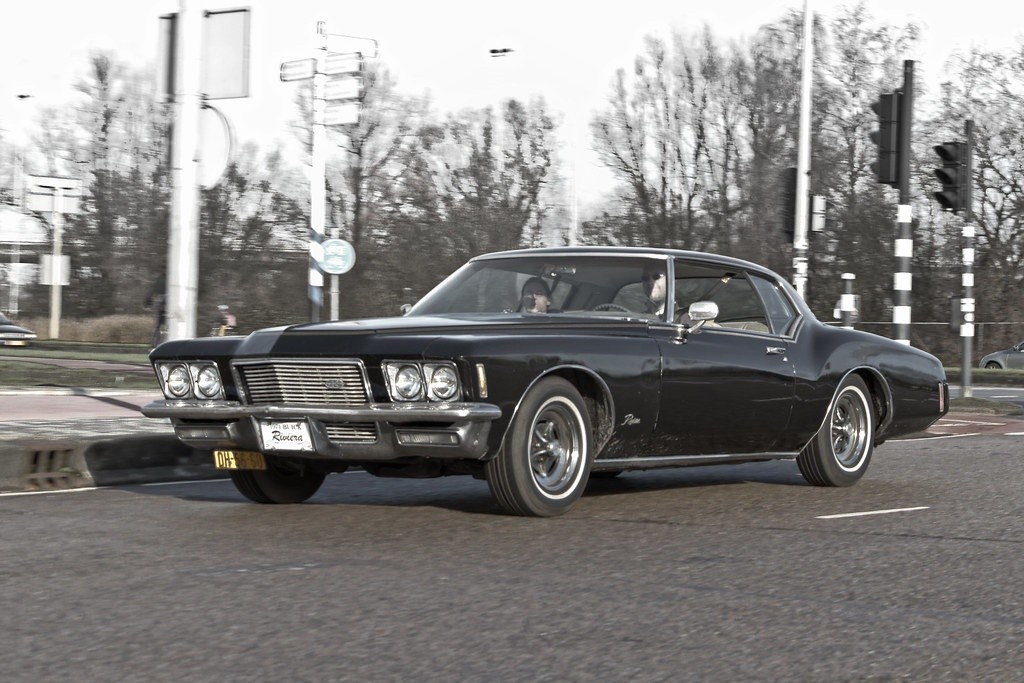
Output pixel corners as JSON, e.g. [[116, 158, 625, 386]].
[[872, 90, 904, 187], [934, 139, 969, 209]]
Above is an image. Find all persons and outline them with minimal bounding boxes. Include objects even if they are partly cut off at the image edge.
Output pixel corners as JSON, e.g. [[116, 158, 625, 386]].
[[520, 277, 562, 313], [143, 267, 236, 337], [640, 261, 701, 327]]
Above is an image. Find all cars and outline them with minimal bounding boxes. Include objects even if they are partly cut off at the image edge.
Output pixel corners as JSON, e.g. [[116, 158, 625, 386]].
[[979, 340, 1024, 372], [158, 246, 953, 518], [0, 312, 37, 348]]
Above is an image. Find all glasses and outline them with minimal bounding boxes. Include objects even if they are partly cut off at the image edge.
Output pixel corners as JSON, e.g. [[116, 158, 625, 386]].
[[640, 273, 666, 281]]
